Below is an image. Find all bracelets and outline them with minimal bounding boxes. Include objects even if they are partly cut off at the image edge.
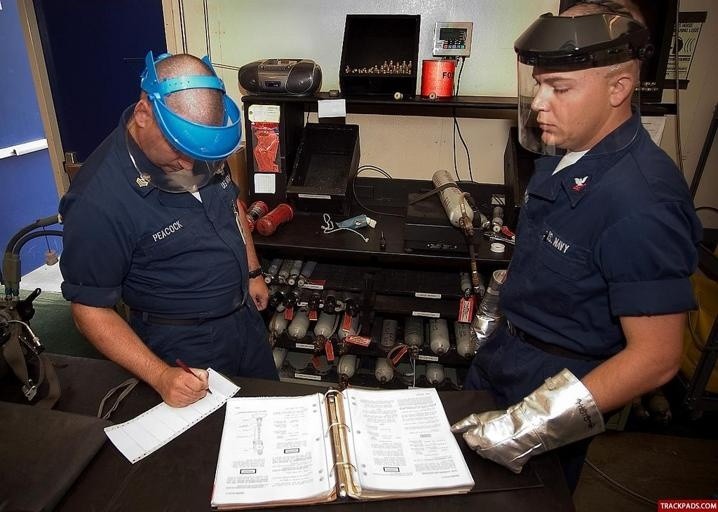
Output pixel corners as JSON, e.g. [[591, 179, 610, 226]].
[[247, 267, 264, 280]]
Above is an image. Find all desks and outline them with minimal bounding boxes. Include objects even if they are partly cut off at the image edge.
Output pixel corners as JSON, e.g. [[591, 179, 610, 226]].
[[41, 353, 566, 512]]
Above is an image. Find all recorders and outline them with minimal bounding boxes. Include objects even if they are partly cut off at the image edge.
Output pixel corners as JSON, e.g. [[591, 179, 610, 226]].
[[238, 59, 322, 97]]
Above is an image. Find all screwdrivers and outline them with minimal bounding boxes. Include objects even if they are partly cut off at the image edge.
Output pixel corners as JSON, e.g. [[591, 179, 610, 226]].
[[380, 231, 386, 249]]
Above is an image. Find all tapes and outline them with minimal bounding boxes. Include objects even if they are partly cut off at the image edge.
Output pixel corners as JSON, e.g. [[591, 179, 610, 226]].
[[490, 243, 505, 253]]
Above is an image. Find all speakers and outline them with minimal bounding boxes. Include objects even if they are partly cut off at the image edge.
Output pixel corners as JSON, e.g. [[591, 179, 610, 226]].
[[559, 0, 679, 104]]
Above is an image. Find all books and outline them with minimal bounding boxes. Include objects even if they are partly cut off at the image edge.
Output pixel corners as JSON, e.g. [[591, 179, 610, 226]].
[[206, 389, 477, 509]]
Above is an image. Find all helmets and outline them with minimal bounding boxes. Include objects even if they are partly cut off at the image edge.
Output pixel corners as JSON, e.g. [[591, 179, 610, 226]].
[[513, 9, 653, 157], [123, 48, 242, 193]]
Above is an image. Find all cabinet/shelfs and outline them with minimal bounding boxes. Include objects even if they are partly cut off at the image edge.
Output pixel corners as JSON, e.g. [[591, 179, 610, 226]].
[[242, 95, 559, 387]]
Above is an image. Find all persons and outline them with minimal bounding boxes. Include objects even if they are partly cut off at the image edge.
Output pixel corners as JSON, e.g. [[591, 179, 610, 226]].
[[462, 2, 702, 497], [60, 54, 279, 411]]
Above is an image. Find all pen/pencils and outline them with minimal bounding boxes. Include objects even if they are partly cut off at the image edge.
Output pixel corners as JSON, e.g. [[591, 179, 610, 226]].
[[175, 358, 213, 394]]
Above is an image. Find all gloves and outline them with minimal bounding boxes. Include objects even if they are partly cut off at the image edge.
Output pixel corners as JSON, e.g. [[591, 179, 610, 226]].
[[449, 368, 606, 475], [470, 270, 511, 358]]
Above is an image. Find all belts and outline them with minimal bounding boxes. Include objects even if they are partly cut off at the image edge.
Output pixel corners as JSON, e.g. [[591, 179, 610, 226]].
[[498, 315, 602, 364], [128, 300, 249, 327]]
